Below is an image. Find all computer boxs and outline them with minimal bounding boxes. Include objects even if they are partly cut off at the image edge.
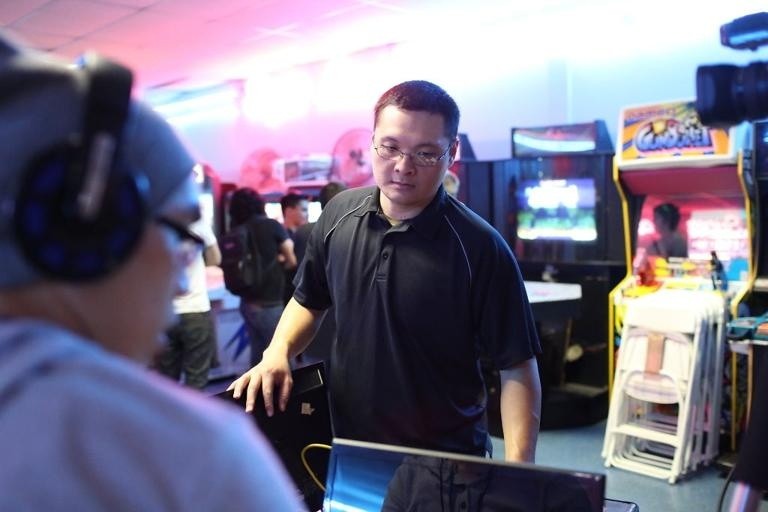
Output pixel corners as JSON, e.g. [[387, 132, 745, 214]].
[[199, 357, 336, 512]]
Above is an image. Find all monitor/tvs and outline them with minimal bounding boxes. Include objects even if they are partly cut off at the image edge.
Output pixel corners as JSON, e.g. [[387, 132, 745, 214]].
[[323, 440, 606, 512], [516, 178, 602, 244]]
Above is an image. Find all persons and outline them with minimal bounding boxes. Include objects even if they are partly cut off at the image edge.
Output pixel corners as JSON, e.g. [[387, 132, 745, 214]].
[[146, 210, 224, 393], [294, 182, 353, 366], [278, 192, 309, 235], [1, 52, 312, 512], [228, 186, 299, 366], [643, 200, 687, 260], [224, 80, 542, 469]]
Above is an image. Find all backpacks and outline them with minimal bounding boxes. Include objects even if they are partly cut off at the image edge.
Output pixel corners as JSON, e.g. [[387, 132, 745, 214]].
[[218, 216, 280, 298]]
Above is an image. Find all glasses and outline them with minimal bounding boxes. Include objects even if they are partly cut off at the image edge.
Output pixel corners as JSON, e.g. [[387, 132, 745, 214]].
[[146, 210, 212, 264], [371, 142, 453, 166]]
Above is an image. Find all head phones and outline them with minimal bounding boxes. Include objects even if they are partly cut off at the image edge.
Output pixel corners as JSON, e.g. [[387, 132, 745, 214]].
[[17, 60, 151, 282]]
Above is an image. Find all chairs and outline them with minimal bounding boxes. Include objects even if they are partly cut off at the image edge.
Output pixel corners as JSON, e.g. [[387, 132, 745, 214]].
[[601, 290, 726, 484]]
